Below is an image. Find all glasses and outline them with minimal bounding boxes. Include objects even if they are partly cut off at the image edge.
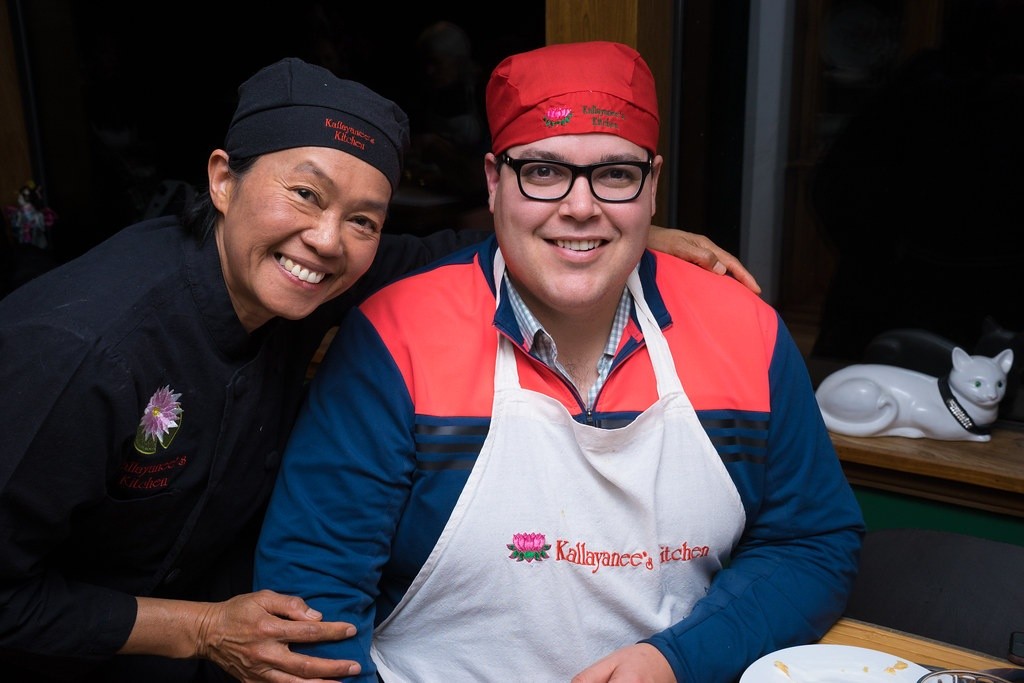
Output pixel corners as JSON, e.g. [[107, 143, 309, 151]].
[[497, 150, 655, 203]]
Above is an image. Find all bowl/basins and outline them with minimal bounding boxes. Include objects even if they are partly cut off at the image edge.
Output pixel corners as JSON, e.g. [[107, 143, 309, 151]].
[[915, 667, 1013, 683]]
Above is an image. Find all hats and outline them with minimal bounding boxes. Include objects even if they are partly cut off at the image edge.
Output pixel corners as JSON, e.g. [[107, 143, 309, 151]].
[[226, 57, 407, 196], [486, 41, 659, 156]]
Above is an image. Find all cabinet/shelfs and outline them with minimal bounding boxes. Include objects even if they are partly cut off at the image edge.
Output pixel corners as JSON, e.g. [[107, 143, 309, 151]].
[[304, 320, 1022, 664]]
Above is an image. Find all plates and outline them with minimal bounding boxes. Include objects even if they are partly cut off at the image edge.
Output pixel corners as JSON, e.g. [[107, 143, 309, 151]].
[[740, 644, 931, 683]]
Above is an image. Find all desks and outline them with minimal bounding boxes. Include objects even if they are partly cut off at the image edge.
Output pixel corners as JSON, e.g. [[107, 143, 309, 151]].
[[819, 616, 1024, 680]]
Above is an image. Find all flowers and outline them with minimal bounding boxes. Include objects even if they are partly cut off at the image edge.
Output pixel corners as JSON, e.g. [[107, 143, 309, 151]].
[[135, 384, 181, 455], [508, 532, 551, 563]]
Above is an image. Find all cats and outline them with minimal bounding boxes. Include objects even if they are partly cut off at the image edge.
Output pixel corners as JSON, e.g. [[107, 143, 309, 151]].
[[815, 346, 1014, 442]]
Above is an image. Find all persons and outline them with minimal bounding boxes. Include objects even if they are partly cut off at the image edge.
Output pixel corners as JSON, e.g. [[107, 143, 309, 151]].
[[0, 57, 762, 683], [250, 41, 868, 683]]
